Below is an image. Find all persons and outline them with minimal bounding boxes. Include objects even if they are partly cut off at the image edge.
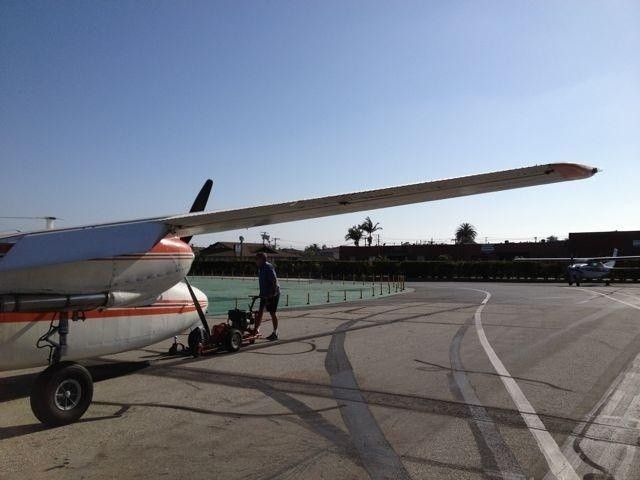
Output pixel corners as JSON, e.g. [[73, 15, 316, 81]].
[[254, 252, 280, 341]]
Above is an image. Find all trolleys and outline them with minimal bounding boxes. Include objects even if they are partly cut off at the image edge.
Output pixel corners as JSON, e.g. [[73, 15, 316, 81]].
[[167, 292, 263, 358]]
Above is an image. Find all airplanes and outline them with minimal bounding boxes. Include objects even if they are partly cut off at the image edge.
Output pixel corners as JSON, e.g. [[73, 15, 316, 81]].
[[515, 248, 640, 288], [2, 161, 603, 429]]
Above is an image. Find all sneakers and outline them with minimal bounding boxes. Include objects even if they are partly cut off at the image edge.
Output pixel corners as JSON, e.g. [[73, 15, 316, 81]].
[[266, 333, 277, 340]]
